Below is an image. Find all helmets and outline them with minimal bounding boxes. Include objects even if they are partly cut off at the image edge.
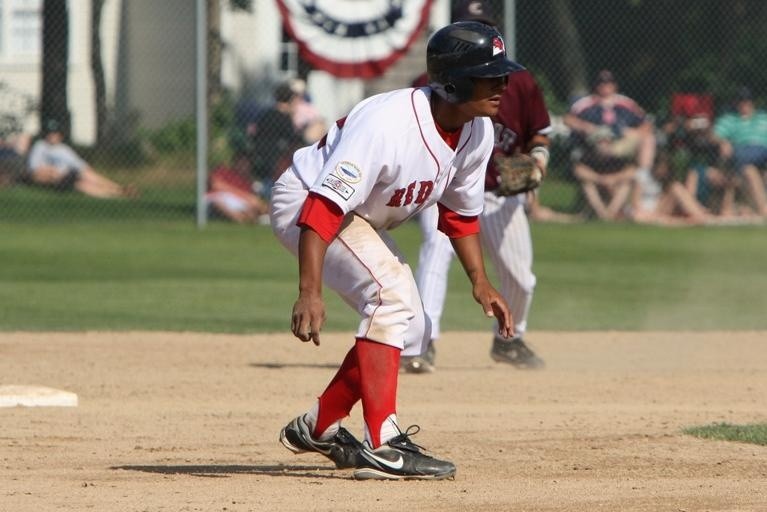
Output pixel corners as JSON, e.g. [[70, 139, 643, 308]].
[[425, 21, 527, 104]]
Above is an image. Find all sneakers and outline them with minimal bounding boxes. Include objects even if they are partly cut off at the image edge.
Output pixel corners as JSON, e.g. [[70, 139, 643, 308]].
[[405, 339, 435, 373], [355, 417, 456, 480], [280, 413, 362, 467], [491, 337, 544, 370]]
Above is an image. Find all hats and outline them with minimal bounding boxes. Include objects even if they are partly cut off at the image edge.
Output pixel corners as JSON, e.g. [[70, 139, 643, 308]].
[[452, 0, 498, 25]]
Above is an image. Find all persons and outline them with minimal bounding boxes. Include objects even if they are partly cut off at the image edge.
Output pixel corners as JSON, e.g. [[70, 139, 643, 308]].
[[266, 20, 529, 482], [525, 70, 765, 226], [208, 70, 324, 227], [403, 19, 551, 374], [26, 118, 142, 202]]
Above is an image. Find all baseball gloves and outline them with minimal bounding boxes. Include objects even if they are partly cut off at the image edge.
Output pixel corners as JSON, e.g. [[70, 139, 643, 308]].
[[494, 154, 543, 198]]
